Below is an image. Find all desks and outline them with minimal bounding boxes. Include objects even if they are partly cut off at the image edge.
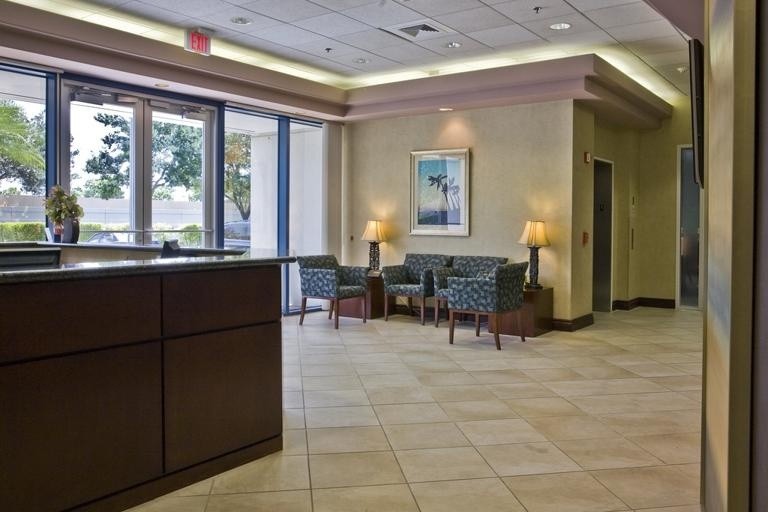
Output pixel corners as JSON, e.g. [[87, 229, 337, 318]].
[[337, 277, 396, 319], [488, 287, 554, 337]]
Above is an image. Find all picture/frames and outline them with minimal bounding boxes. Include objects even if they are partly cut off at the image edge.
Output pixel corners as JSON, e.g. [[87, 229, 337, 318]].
[[409, 146, 470, 237]]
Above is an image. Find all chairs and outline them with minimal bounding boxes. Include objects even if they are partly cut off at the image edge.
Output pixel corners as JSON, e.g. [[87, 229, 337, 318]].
[[296, 254, 370, 328], [447, 261, 530, 350], [382, 253, 452, 325], [432, 256, 508, 327]]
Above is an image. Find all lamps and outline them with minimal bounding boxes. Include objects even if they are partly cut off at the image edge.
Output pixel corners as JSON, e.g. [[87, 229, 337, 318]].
[[361, 221, 387, 278], [517, 221, 551, 289]]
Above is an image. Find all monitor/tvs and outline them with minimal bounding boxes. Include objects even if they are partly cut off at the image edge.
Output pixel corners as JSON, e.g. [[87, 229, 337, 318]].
[[160, 240, 182, 259], [687, 38, 704, 189]]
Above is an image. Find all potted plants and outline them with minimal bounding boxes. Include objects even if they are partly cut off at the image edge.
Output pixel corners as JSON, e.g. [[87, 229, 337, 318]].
[[40, 184, 84, 243]]
